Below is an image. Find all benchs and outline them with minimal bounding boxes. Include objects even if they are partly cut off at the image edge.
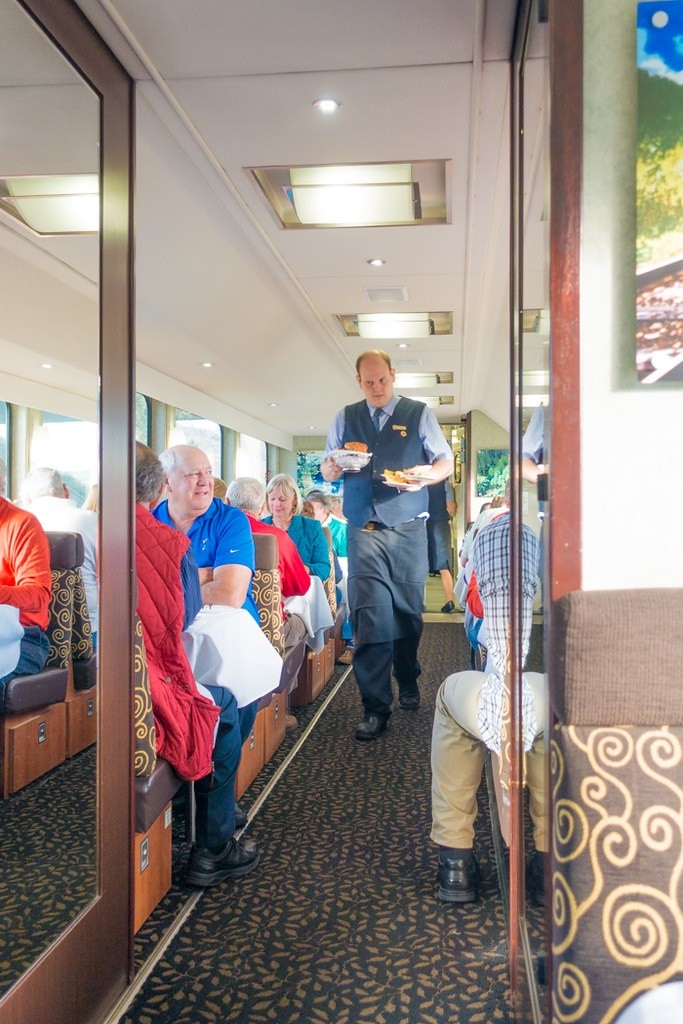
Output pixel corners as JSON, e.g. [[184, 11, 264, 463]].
[[3, 530, 96, 798], [135, 528, 336, 931], [542, 584, 683, 1024]]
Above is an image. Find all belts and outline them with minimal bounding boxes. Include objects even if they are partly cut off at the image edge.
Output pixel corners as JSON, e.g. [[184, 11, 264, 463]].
[[364, 522, 386, 530]]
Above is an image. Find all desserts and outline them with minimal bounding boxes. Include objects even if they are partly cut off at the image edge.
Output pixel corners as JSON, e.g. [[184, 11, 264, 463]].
[[380, 469, 406, 483]]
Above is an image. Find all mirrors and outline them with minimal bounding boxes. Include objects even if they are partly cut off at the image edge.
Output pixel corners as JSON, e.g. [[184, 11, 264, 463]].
[[507, 0, 554, 1024], [0, 0, 133, 1024]]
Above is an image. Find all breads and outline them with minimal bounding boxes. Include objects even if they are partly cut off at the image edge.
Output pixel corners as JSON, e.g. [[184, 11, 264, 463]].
[[344, 442, 368, 453]]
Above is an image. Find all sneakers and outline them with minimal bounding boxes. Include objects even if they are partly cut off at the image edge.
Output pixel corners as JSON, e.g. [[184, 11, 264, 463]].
[[233, 802, 247, 827], [337, 649, 355, 664], [285, 715, 298, 731]]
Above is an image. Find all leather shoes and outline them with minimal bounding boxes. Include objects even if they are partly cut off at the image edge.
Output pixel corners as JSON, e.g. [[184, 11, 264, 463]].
[[442, 601, 455, 613], [184, 837, 261, 888], [398, 694, 420, 709], [354, 715, 387, 740], [437, 849, 480, 902]]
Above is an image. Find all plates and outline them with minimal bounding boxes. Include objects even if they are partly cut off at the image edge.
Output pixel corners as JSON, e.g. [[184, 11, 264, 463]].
[[381, 481, 419, 489], [404, 464, 432, 474], [400, 475, 437, 483], [379, 474, 404, 483]]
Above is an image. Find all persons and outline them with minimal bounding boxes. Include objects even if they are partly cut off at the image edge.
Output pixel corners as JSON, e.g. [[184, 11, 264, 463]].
[[257, 474, 331, 582], [15, 467, 98, 652], [135, 441, 260, 887], [224, 477, 311, 733], [324, 350, 455, 740], [150, 445, 282, 827], [300, 489, 355, 665], [520, 400, 546, 612], [0, 460, 52, 691], [427, 479, 508, 901], [521, 523, 548, 751]]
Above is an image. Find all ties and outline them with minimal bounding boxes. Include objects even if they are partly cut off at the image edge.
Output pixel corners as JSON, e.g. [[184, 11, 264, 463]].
[[371, 408, 383, 431]]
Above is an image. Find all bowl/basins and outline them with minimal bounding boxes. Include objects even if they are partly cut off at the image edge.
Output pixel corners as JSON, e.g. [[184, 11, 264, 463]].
[[333, 449, 371, 473]]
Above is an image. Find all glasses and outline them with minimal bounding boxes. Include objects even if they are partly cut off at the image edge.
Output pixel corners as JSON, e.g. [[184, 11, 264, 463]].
[[332, 499, 340, 504]]
